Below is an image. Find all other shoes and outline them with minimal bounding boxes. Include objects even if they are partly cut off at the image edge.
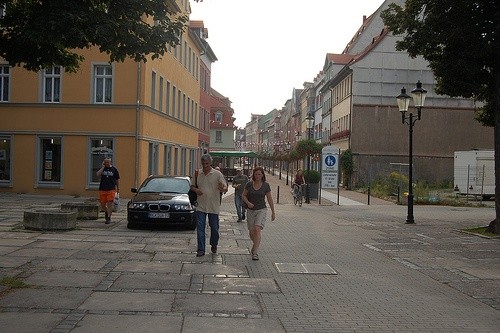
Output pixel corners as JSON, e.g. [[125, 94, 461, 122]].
[[237, 218, 242, 222], [211, 244, 217, 253], [242, 214, 245, 220], [105, 212, 108, 219], [105, 217, 110, 224], [196, 250, 205, 257]]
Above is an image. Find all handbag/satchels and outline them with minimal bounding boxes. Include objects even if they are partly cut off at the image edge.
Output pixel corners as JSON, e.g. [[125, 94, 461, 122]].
[[188, 169, 199, 207], [241, 182, 253, 209], [113, 192, 120, 210]]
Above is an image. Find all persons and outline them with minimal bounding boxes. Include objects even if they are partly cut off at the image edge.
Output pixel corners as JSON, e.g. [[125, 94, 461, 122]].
[[242, 167, 275, 260], [191, 154, 228, 257], [232, 167, 248, 222], [97, 157, 120, 224], [294, 169, 305, 200]]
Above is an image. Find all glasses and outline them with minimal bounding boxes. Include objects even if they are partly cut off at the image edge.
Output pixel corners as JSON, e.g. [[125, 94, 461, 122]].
[[104, 157, 109, 159], [203, 161, 210, 165]]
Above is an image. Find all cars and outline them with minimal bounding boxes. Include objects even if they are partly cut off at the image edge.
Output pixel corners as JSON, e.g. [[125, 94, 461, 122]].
[[126, 175, 196, 230]]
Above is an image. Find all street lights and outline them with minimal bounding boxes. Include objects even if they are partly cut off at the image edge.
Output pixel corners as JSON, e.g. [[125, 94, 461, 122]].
[[286, 141, 291, 185], [396, 79, 428, 224], [295, 131, 302, 170], [303, 113, 315, 204], [279, 144, 283, 179], [272, 147, 276, 176]]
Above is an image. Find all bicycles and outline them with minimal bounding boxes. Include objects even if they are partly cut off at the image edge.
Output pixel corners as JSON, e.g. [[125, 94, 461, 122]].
[[291, 182, 309, 207]]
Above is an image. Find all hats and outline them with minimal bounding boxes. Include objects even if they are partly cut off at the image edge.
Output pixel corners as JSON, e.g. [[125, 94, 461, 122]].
[[236, 167, 242, 171]]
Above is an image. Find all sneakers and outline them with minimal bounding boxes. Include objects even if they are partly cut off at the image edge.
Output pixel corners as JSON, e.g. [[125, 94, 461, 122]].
[[252, 251, 259, 260]]
